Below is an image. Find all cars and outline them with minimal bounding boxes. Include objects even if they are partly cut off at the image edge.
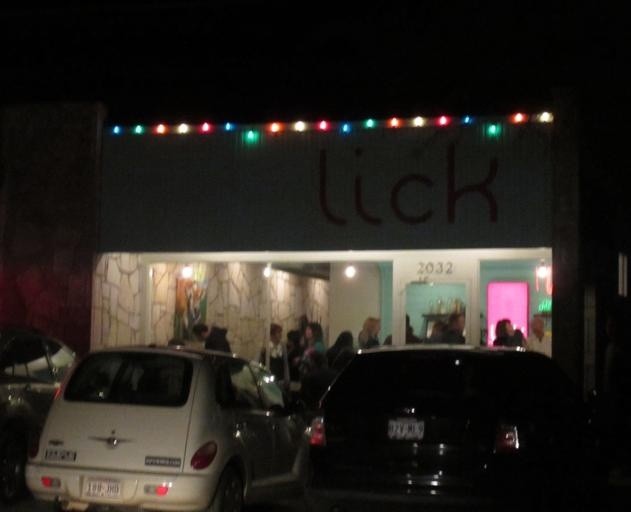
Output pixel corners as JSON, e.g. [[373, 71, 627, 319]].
[[0, 327, 81, 504], [303, 346, 609, 512], [25, 343, 311, 512]]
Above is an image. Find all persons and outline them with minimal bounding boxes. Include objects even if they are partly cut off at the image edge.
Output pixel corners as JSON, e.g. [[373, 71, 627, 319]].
[[258, 313, 552, 424], [192, 321, 231, 352]]
